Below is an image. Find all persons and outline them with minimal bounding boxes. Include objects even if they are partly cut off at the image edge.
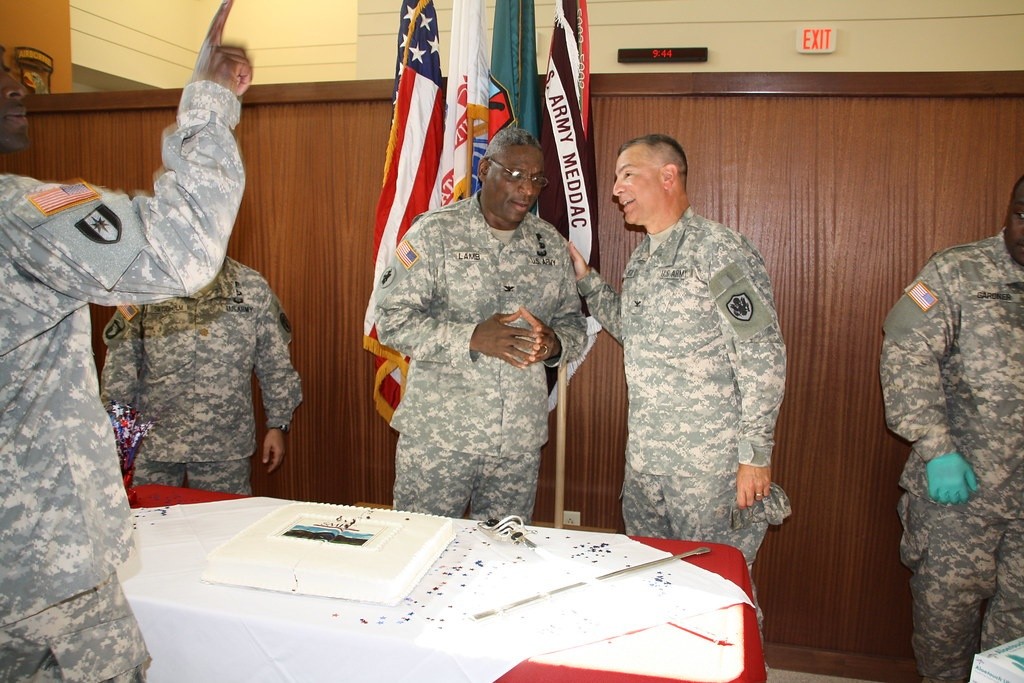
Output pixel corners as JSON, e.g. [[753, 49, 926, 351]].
[[570, 132, 786, 675], [377, 129, 590, 526], [99, 256, 304, 497], [877, 179, 1024, 683], [0, 0, 254, 683]]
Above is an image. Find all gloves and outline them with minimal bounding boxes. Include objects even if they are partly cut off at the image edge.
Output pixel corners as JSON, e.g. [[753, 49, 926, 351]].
[[925, 452, 978, 506]]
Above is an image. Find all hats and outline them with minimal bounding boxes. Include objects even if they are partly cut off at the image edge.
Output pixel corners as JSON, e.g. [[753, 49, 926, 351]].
[[728, 482, 793, 532]]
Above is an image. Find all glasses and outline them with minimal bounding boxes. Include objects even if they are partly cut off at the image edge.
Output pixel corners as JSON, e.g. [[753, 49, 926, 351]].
[[487, 157, 549, 187]]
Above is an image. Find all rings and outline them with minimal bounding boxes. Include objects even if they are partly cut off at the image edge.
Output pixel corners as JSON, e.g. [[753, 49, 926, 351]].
[[544, 345, 548, 355], [756, 493, 762, 496]]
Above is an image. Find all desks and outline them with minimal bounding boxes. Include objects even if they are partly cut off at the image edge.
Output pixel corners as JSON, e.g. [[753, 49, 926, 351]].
[[124, 481, 772, 683]]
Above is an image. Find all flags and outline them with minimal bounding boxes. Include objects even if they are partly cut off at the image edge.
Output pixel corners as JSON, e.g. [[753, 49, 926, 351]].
[[363, 0, 603, 424]]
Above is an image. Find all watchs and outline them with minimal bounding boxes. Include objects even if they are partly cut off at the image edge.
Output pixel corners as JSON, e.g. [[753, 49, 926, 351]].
[[270, 425, 290, 433]]
